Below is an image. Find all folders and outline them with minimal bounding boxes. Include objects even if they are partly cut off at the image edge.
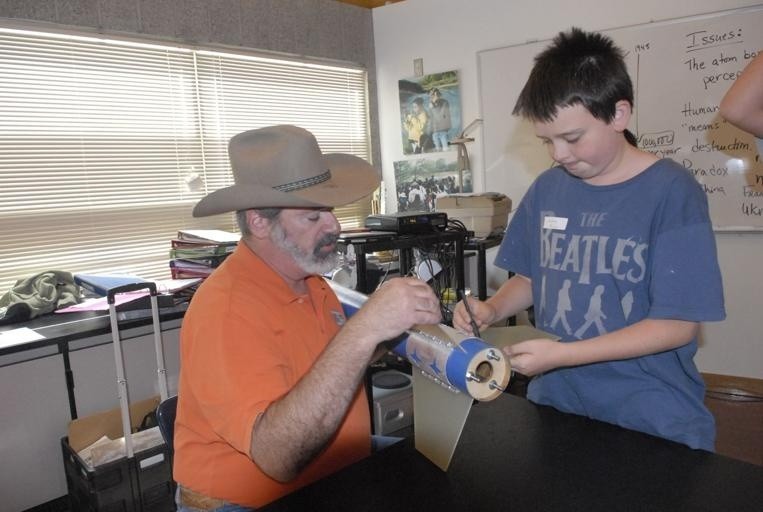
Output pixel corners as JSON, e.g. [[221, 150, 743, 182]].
[[169, 230, 242, 279], [74, 275, 157, 297]]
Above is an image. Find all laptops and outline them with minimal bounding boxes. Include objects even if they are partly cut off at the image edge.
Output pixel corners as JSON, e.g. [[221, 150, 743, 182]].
[[338, 231, 397, 243]]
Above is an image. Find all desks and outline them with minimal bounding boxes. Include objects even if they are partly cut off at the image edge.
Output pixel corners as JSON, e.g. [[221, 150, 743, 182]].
[[252, 391, 763, 512], [1, 301, 188, 423]]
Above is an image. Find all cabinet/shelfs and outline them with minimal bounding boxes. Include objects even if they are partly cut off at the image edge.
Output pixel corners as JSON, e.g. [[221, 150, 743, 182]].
[[334, 229, 518, 437]]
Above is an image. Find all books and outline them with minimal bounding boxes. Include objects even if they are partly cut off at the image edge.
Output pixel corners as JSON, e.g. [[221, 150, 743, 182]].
[[435, 192, 512, 238]]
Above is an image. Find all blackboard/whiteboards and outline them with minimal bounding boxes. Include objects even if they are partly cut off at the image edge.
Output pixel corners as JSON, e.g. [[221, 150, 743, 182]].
[[476, 4, 763, 233]]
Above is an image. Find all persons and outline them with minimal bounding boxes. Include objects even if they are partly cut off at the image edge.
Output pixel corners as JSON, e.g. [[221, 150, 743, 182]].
[[720, 50, 763, 139], [452, 28, 726, 454], [173, 125, 442, 512], [404, 87, 451, 154], [397, 176, 456, 213]]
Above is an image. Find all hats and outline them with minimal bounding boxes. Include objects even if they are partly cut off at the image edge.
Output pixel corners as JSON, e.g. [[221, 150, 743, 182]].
[[191, 124, 382, 217]]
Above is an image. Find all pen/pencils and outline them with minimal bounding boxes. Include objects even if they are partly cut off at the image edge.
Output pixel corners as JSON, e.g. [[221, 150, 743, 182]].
[[458, 289, 480, 338]]
